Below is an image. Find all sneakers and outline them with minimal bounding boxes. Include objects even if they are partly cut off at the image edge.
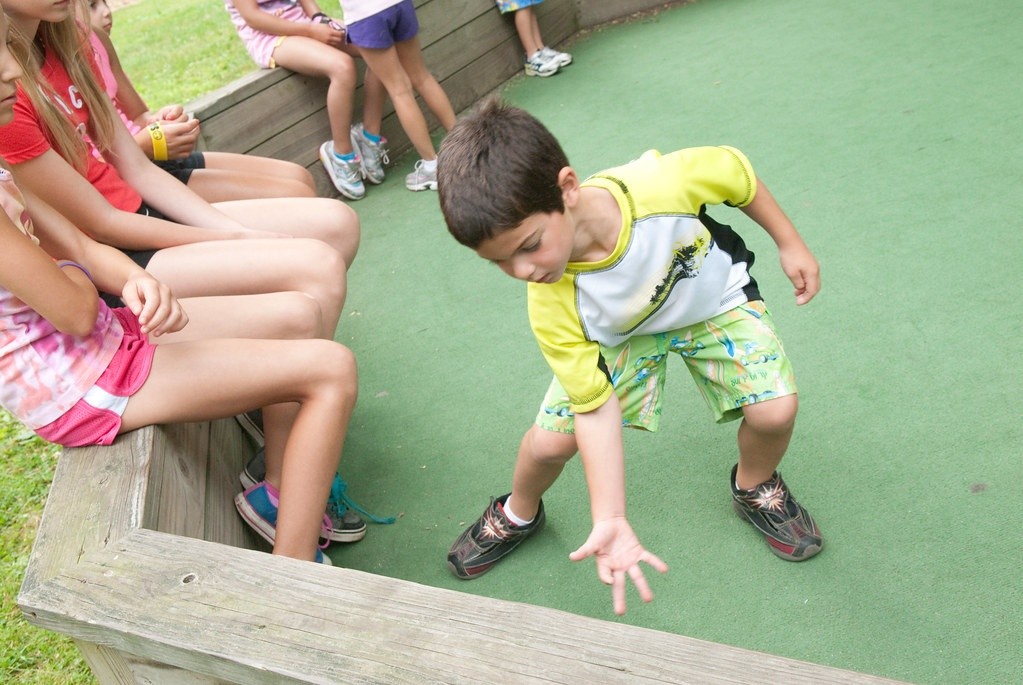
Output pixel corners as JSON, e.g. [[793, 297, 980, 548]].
[[524, 54, 560, 77], [538, 46, 573, 68], [447, 493, 546, 579], [405, 164, 439, 191], [234, 408, 266, 448], [349, 121, 388, 185], [240, 446, 367, 543], [730, 461, 824, 562], [318, 139, 366, 200], [234, 480, 333, 570]]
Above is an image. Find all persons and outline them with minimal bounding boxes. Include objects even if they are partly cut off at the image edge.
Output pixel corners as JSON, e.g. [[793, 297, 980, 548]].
[[222, 0, 387, 201], [435, 98, 826, 616], [63, 0, 319, 203], [0, 1, 385, 546], [491, 0, 573, 78], [0, 5, 361, 579], [338, 0, 467, 192]]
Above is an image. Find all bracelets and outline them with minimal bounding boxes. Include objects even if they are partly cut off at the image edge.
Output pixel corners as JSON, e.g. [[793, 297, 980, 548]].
[[311, 12, 327, 21], [148, 121, 169, 161], [57, 262, 93, 282]]
